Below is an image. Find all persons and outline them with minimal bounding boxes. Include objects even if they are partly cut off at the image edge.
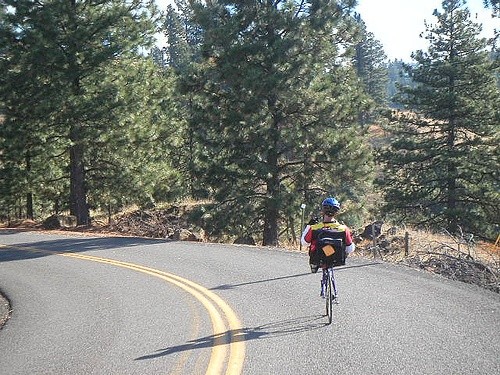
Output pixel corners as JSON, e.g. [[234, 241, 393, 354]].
[[300, 198, 355, 255]]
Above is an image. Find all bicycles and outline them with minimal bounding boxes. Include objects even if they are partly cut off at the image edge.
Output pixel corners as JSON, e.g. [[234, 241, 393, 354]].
[[319, 265, 338, 325]]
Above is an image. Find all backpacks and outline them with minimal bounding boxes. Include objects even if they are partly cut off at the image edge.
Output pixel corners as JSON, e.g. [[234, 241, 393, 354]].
[[313, 226, 346, 265]]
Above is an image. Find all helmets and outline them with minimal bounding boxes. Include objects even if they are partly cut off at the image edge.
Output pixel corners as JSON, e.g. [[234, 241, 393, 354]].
[[322, 198, 341, 216]]
